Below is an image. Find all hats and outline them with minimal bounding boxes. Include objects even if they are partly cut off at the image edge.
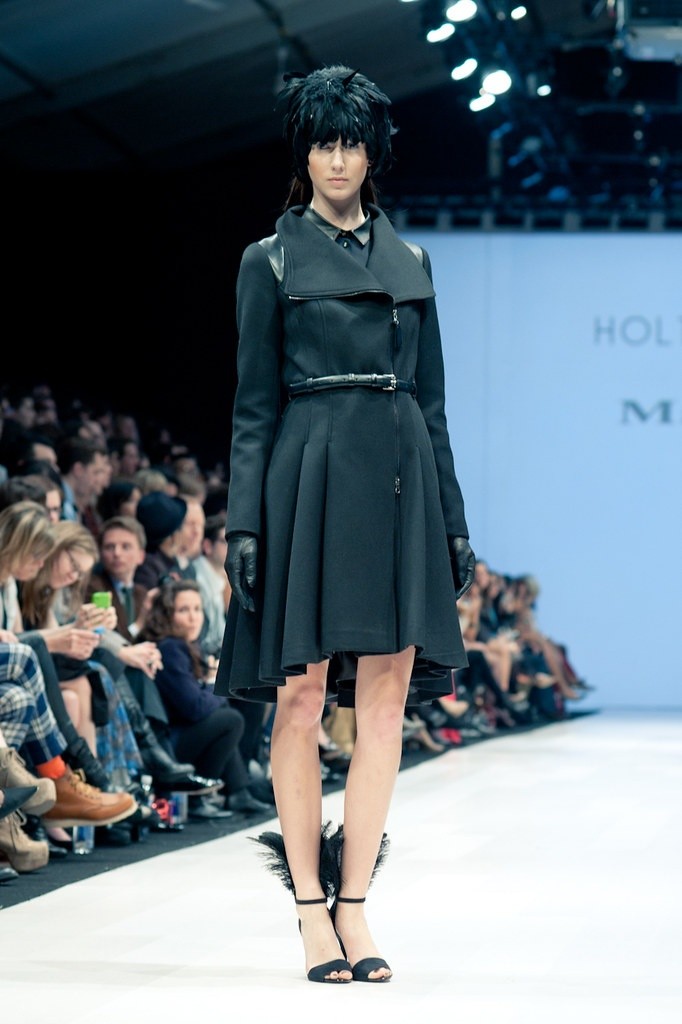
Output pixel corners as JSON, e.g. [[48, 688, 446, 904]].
[[135, 491, 188, 540]]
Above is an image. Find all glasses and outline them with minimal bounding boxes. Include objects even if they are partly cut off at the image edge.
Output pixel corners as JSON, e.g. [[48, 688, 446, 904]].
[[63, 547, 87, 582]]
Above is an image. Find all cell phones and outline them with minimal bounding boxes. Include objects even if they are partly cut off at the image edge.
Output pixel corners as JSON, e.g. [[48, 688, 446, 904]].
[[93, 627, 104, 634], [91, 592, 111, 609]]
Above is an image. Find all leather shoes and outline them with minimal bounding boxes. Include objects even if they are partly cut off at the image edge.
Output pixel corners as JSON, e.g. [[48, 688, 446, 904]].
[[40, 763, 140, 828], [20, 815, 69, 856], [0, 786, 38, 819]]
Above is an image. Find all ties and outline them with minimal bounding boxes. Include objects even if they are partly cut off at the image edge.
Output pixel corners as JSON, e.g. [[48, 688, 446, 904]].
[[122, 586, 134, 628]]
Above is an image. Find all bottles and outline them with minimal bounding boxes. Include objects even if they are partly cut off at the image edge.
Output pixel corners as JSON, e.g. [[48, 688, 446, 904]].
[[141, 775, 154, 834]]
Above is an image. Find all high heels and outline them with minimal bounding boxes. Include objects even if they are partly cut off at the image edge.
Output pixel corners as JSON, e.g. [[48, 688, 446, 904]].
[[0, 809, 49, 872], [0, 747, 56, 816], [329, 899, 393, 982], [294, 898, 353, 983]]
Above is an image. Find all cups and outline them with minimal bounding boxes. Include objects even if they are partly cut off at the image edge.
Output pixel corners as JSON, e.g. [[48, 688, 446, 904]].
[[93, 593, 111, 609], [170, 793, 187, 829], [73, 825, 93, 854]]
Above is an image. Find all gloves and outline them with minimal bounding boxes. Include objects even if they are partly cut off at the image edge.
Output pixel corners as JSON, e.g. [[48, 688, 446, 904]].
[[449, 536, 476, 599], [224, 532, 255, 613]]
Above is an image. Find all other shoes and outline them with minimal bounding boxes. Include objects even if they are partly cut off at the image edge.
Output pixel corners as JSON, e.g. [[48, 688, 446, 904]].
[[47, 834, 72, 848], [124, 677, 595, 842], [94, 825, 131, 846]]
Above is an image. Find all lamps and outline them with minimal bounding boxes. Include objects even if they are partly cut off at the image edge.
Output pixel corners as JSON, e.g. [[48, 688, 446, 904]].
[[420, 0, 513, 113]]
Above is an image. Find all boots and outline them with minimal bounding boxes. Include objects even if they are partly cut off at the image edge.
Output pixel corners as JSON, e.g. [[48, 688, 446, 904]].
[[68, 738, 143, 824]]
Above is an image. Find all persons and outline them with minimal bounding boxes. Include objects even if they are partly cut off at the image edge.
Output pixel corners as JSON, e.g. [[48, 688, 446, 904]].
[[224, 65, 475, 982], [0, 384, 279, 883], [318, 557, 594, 783]]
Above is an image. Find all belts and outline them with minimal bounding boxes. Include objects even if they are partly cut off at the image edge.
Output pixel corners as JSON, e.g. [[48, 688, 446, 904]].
[[288, 372, 416, 397]]
